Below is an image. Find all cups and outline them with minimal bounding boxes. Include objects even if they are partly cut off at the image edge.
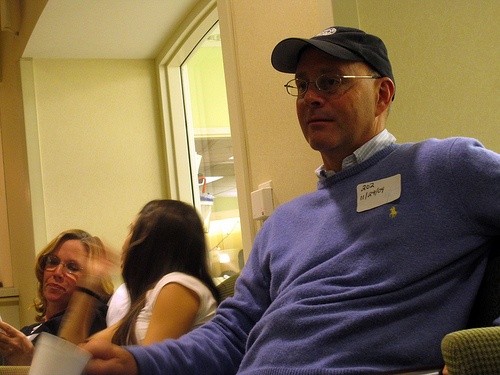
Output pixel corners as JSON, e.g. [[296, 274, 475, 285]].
[[28, 332, 93, 375]]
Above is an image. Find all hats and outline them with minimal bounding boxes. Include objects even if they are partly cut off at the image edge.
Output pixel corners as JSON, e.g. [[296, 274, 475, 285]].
[[271, 25, 396, 101]]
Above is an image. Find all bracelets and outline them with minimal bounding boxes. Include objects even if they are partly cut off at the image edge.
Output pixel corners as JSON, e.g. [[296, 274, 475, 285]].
[[74, 285, 101, 301]]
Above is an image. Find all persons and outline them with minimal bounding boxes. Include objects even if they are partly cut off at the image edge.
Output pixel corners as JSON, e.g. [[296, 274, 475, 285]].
[[1, 198, 222, 367], [75, 25, 500, 375], [0, 228, 115, 344]]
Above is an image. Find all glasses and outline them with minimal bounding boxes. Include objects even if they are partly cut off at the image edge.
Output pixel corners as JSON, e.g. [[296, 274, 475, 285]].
[[284, 73, 384, 96], [44, 255, 84, 275]]
